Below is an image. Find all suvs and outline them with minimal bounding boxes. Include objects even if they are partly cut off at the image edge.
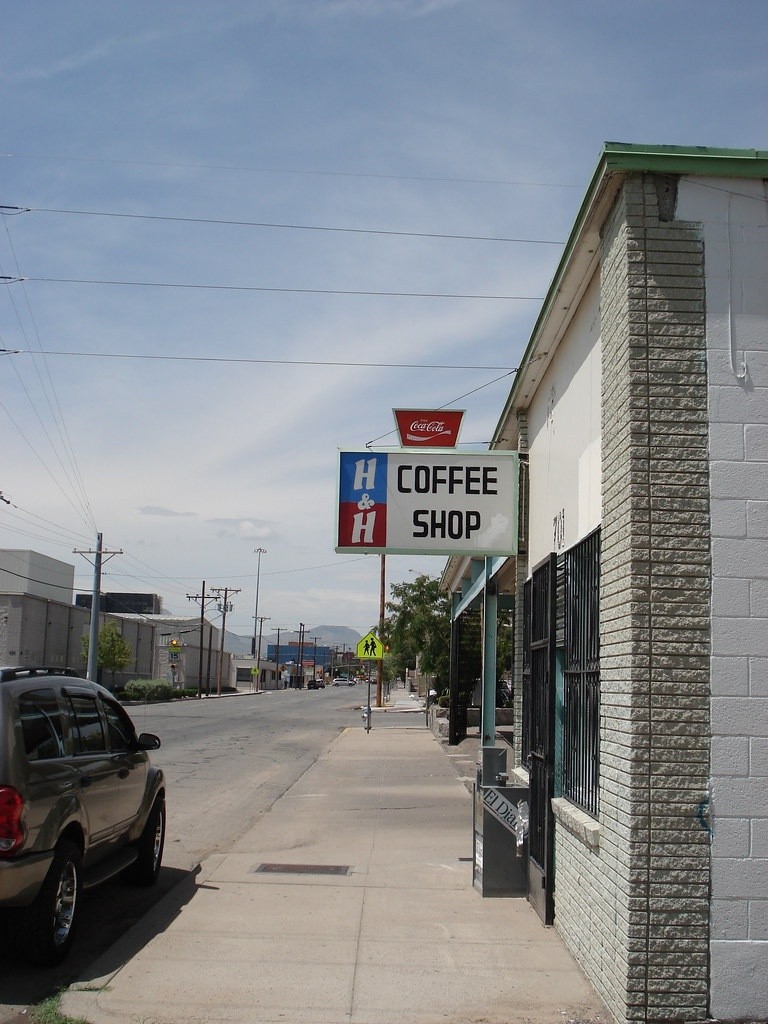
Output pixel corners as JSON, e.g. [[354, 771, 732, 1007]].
[[331, 678, 356, 687], [1, 662, 167, 971]]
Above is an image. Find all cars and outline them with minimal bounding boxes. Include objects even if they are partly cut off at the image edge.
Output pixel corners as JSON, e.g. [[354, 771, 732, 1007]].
[[308, 679, 325, 689], [354, 676, 377, 684]]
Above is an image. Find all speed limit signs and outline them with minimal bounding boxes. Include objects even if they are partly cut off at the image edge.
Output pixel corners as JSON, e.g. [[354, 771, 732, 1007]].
[[169, 651, 180, 661]]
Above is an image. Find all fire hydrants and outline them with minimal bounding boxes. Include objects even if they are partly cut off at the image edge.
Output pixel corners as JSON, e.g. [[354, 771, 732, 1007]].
[[363, 705, 373, 730]]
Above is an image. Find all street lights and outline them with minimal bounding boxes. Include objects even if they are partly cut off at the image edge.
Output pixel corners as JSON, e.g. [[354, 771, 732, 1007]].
[[252, 547, 267, 658], [271, 628, 287, 690], [309, 636, 322, 680]]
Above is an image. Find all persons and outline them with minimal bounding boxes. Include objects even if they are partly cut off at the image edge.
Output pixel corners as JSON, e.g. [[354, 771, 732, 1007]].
[[284, 679, 288, 689]]
